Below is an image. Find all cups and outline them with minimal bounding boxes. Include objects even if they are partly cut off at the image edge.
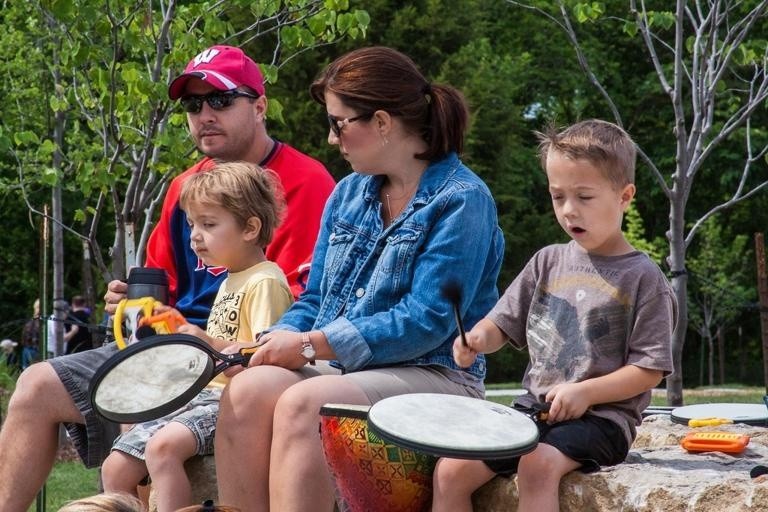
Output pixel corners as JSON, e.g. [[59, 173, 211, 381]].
[[326, 112, 371, 137], [181, 88, 258, 112]]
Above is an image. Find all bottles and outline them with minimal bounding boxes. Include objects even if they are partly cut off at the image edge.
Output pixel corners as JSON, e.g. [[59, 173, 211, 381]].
[[169, 45, 265, 100], [1, 339, 17, 347]]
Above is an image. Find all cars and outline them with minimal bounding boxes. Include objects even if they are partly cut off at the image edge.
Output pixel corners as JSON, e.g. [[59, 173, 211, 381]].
[[123, 267, 169, 307]]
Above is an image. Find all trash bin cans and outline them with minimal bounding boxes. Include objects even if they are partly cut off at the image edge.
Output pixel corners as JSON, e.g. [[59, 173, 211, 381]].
[[297, 332, 318, 362]]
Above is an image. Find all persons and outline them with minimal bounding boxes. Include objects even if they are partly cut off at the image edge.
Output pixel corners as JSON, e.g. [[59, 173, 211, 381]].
[[98, 163, 295, 512], [0, 292, 101, 372], [432, 120, 674, 512], [215, 46, 507, 511], [53, 489, 149, 512], [0, 44, 337, 512]]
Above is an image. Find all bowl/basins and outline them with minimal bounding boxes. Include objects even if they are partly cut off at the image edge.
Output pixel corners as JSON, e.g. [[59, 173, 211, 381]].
[[380, 171, 427, 227]]
